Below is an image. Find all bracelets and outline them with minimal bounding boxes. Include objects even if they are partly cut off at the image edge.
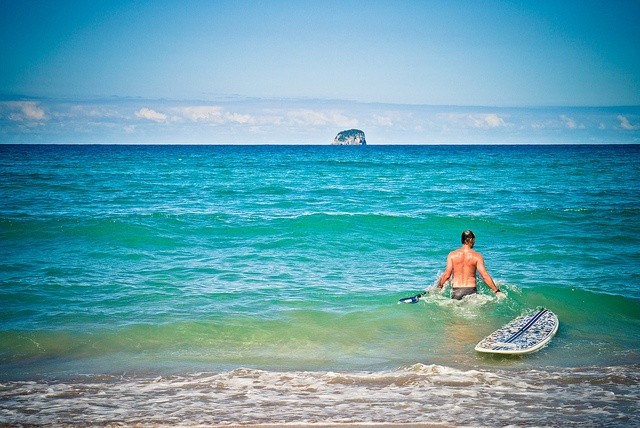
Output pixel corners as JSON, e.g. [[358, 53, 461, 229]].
[[495, 288, 501, 294]]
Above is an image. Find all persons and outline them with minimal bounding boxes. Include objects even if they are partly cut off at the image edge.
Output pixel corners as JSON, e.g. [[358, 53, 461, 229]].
[[437, 229, 507, 302]]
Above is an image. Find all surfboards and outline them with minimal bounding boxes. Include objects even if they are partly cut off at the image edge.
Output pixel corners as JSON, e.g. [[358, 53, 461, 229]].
[[475, 306, 559, 354]]
